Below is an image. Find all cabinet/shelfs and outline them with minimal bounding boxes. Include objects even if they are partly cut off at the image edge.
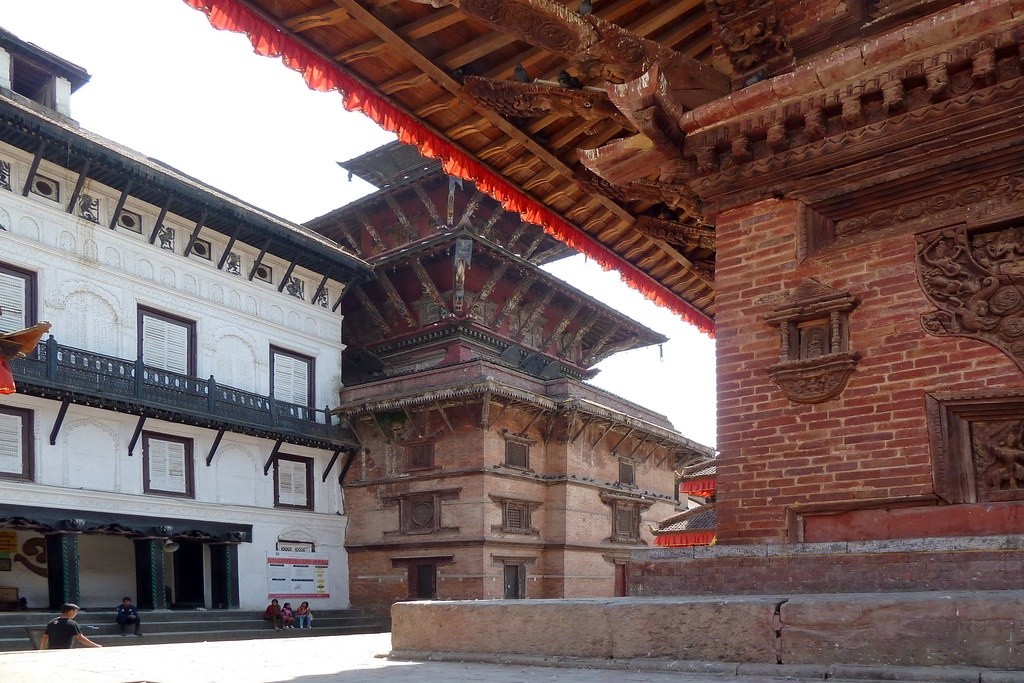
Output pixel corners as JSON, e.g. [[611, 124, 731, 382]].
[[0, 586, 19, 601]]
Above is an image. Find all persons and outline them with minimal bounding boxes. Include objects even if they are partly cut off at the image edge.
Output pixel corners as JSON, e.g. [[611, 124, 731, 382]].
[[264, 599, 312, 632], [115, 597, 143, 637], [40, 602, 102, 650]]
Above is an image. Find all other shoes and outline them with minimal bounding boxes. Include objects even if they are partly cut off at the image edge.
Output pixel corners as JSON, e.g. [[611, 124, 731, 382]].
[[308, 625, 311, 628], [290, 625, 294, 629], [121, 632, 125, 636], [283, 627, 287, 630], [286, 626, 289, 629], [300, 625, 303, 628], [135, 632, 142, 636], [274, 626, 279, 630]]
